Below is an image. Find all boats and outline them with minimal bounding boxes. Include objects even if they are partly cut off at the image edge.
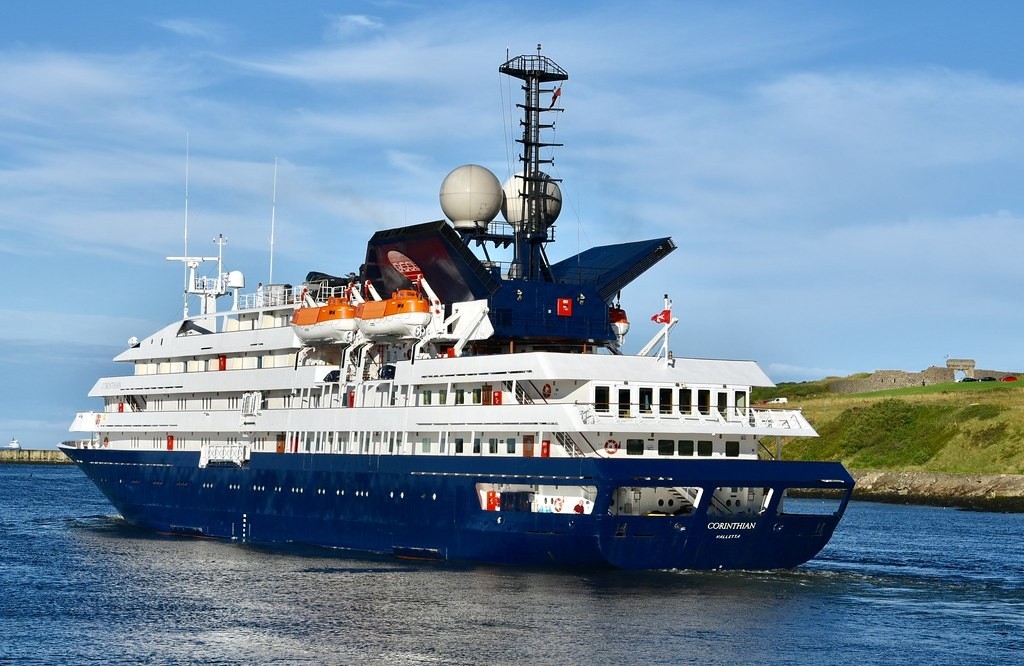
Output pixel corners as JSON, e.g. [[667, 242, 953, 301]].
[[608, 308, 630, 336], [358, 273, 431, 337], [290, 281, 359, 343], [57, 46, 856, 570]]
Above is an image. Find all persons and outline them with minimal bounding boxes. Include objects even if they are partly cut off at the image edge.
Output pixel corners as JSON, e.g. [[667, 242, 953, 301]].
[[573, 500, 585, 514], [257, 283, 264, 308]]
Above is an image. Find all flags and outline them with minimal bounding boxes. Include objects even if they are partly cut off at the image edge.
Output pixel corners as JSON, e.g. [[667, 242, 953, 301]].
[[650, 309, 671, 324]]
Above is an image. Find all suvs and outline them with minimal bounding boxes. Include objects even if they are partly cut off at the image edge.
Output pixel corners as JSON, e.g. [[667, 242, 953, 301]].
[[766, 397, 788, 403]]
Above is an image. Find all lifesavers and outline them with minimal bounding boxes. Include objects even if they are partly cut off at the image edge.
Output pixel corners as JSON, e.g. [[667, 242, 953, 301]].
[[554, 498, 562, 512], [543, 384, 551, 398], [436, 351, 444, 358], [605, 440, 618, 454], [104, 437, 108, 447]]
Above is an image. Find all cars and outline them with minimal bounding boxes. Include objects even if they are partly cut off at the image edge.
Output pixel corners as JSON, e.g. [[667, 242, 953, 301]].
[[958, 376, 1018, 382]]
